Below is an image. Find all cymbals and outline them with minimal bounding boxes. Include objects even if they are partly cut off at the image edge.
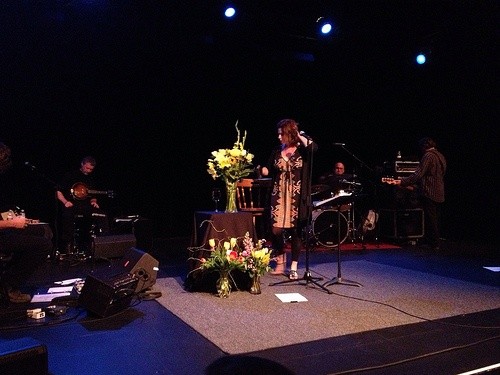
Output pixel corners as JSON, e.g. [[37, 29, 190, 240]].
[[250, 177, 275, 185]]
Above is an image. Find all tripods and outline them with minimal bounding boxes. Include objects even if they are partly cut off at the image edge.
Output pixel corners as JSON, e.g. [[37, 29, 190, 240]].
[[269, 140, 363, 294]]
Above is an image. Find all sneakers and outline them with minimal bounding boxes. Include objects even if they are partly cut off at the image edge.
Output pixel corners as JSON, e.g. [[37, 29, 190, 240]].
[[8, 286, 31, 303]]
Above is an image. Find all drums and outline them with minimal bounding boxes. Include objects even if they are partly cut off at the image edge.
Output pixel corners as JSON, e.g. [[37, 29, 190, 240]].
[[307, 181, 360, 248]]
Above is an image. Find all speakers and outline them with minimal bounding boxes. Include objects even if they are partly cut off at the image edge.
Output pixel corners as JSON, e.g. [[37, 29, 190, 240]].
[[77, 233, 160, 318], [386, 176, 421, 210], [0, 344, 49, 375]]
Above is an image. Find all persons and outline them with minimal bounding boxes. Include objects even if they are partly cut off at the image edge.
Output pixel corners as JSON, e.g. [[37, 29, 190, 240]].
[[321, 162, 350, 190], [0, 142, 53, 302], [256, 119, 316, 280], [55, 157, 100, 247], [400, 138, 446, 250]]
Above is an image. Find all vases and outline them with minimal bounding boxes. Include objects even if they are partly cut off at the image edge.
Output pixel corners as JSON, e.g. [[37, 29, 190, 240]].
[[250, 270, 262, 294], [225, 178, 238, 213], [217, 269, 231, 297]]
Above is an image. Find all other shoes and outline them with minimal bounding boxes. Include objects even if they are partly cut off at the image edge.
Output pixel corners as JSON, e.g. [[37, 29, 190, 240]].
[[270, 267, 285, 274], [432, 246, 440, 256], [289, 270, 298, 280]]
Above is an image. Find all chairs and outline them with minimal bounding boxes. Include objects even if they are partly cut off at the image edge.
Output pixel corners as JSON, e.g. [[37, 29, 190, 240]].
[[236, 179, 272, 227]]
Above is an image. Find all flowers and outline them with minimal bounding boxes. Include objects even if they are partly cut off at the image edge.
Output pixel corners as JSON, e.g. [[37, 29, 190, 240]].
[[186, 220, 273, 297], [207, 120, 255, 211]]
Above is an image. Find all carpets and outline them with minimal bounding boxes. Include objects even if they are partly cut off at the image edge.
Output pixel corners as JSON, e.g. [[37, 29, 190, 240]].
[[283, 241, 402, 253], [145, 260, 500, 355]]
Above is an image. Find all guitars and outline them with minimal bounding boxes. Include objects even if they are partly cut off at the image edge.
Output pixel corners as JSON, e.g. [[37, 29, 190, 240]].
[[71, 182, 114, 204], [380, 176, 414, 190]]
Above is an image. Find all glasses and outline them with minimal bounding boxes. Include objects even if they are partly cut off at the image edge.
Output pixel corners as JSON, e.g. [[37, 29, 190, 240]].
[[277, 133, 283, 136]]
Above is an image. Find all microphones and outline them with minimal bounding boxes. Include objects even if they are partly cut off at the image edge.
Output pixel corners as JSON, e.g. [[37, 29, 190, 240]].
[[334, 143, 346, 145], [300, 130, 313, 140]]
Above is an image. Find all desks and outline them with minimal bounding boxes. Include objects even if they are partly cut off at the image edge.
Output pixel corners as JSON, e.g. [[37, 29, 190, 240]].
[[191, 211, 258, 261]]
